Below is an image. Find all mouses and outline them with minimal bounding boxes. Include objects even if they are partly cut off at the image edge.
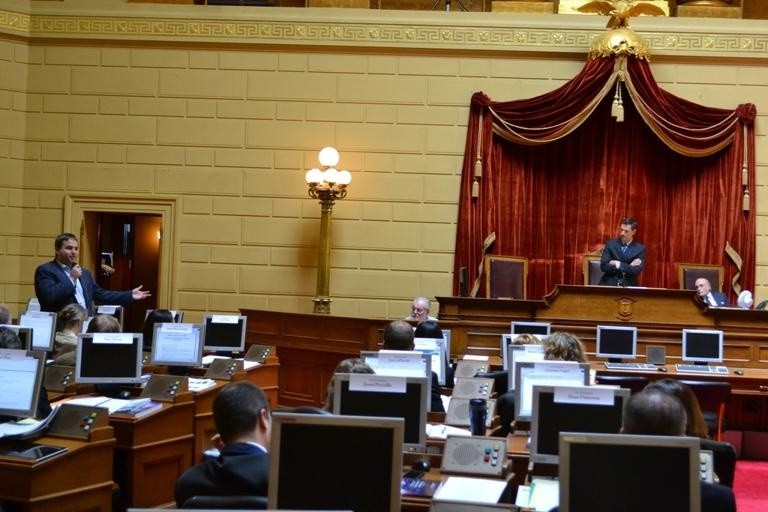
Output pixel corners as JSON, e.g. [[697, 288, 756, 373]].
[[736, 370, 743, 375]]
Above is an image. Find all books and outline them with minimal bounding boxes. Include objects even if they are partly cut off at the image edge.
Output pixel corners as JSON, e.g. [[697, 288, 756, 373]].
[[399, 477, 444, 499], [516, 478, 561, 511], [0, 402, 64, 436], [109, 400, 163, 418], [425, 422, 472, 443], [188, 376, 216, 391], [65, 396, 152, 414]]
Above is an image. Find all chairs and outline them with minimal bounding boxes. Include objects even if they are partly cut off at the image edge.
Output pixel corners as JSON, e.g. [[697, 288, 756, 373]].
[[677, 262, 725, 292], [484, 255, 530, 300], [277, 355, 766, 511], [581, 245, 611, 288]]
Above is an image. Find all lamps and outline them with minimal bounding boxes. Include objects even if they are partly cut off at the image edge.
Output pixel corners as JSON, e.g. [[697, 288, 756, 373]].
[[302, 145, 353, 313]]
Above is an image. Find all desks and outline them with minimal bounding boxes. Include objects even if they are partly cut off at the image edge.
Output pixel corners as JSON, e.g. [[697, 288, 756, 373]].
[[1, 348, 278, 511]]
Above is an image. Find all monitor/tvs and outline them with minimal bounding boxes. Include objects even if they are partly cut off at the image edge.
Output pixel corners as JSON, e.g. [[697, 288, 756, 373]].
[[412, 328, 451, 364], [682, 329, 722, 363], [266, 410, 402, 512], [595, 324, 638, 359], [507, 344, 547, 393], [515, 362, 590, 422], [412, 336, 446, 386], [333, 373, 428, 455], [511, 320, 550, 335], [359, 350, 431, 414], [501, 333, 550, 370], [558, 432, 702, 512], [0, 296, 247, 418], [531, 384, 630, 465]]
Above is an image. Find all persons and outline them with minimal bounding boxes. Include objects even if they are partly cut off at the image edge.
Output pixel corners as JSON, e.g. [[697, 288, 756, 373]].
[[552, 395, 737, 511], [404, 298, 440, 325], [34, 233, 153, 332], [140, 308, 174, 350], [50, 302, 88, 351], [322, 358, 377, 414], [53, 315, 122, 366], [414, 323, 454, 385], [0, 305, 13, 326], [176, 378, 276, 510], [0, 326, 55, 419], [694, 277, 731, 307], [381, 320, 447, 412], [597, 217, 645, 286], [641, 378, 712, 439], [494, 333, 598, 437], [511, 333, 540, 347]]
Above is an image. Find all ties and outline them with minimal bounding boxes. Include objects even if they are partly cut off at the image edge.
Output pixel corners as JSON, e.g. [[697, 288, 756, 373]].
[[704, 296, 712, 307], [617, 245, 627, 284]]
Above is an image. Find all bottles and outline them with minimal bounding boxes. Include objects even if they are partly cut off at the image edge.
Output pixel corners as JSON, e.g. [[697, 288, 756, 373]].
[[469, 398, 487, 435]]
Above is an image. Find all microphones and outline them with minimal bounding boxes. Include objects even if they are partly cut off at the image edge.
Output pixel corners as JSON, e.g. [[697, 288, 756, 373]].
[[621, 267, 626, 286], [70, 261, 81, 282]]
